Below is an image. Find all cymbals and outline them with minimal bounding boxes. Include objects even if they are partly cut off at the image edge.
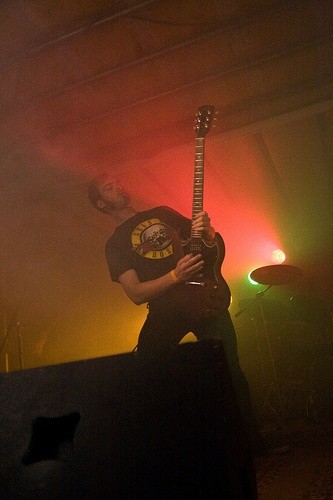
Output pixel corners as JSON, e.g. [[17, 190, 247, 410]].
[[238, 298, 287, 318], [250, 265, 304, 286]]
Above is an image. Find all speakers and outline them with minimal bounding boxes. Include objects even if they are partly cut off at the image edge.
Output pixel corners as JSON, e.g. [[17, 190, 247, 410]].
[[0, 339, 260, 500]]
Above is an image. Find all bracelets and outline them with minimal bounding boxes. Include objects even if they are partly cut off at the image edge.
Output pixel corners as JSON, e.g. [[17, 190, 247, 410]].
[[202, 238, 217, 248], [171, 270, 179, 283]]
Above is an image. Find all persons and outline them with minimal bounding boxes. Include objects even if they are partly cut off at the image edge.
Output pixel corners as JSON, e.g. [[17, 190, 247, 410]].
[[90, 172, 290, 456]]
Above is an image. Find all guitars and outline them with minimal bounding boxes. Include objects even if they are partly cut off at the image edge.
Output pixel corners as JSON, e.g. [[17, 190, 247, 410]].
[[173, 104, 232, 323]]
